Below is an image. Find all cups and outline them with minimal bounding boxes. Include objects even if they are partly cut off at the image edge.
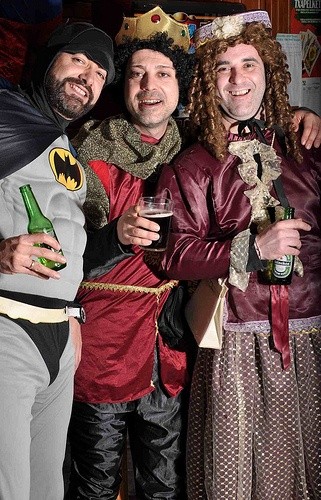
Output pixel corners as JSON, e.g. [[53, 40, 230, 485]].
[[137, 196, 173, 251]]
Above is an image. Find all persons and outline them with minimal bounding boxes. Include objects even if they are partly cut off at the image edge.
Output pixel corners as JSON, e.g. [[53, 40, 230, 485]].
[[70, 5, 321, 500], [0, 16, 115, 500], [140, 10, 321, 500]]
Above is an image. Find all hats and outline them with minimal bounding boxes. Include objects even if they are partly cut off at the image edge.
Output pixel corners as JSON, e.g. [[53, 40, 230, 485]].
[[193, 11, 272, 50], [114, 5, 190, 51]]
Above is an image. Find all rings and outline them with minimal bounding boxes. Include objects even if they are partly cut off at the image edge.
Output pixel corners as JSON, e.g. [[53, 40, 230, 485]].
[[28, 259, 36, 269]]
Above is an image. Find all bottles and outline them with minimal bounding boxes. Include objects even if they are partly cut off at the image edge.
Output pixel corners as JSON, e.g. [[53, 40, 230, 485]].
[[271, 206, 296, 286], [265, 206, 285, 282], [19, 184, 68, 272]]
